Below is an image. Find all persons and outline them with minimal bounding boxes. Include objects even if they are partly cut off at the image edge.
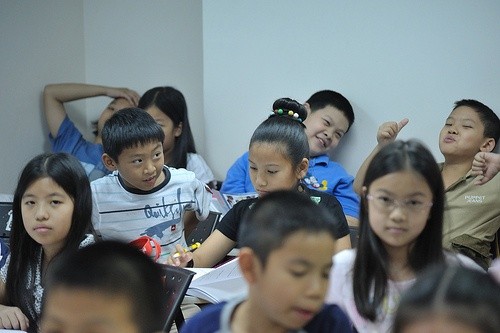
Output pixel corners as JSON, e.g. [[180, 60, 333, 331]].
[[0, 83, 500, 333]]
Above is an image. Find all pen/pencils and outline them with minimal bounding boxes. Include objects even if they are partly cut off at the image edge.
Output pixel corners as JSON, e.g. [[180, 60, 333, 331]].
[[173, 242, 201, 258]]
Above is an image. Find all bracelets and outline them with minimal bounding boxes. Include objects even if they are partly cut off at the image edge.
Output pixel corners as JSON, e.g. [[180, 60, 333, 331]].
[[187, 258, 194, 268]]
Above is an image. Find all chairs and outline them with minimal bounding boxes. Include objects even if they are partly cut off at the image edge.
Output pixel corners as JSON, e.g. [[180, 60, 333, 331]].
[[183, 206, 222, 258], [145, 262, 197, 333]]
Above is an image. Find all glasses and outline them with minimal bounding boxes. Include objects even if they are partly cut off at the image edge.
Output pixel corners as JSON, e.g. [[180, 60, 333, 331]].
[[365, 194, 434, 211]]
